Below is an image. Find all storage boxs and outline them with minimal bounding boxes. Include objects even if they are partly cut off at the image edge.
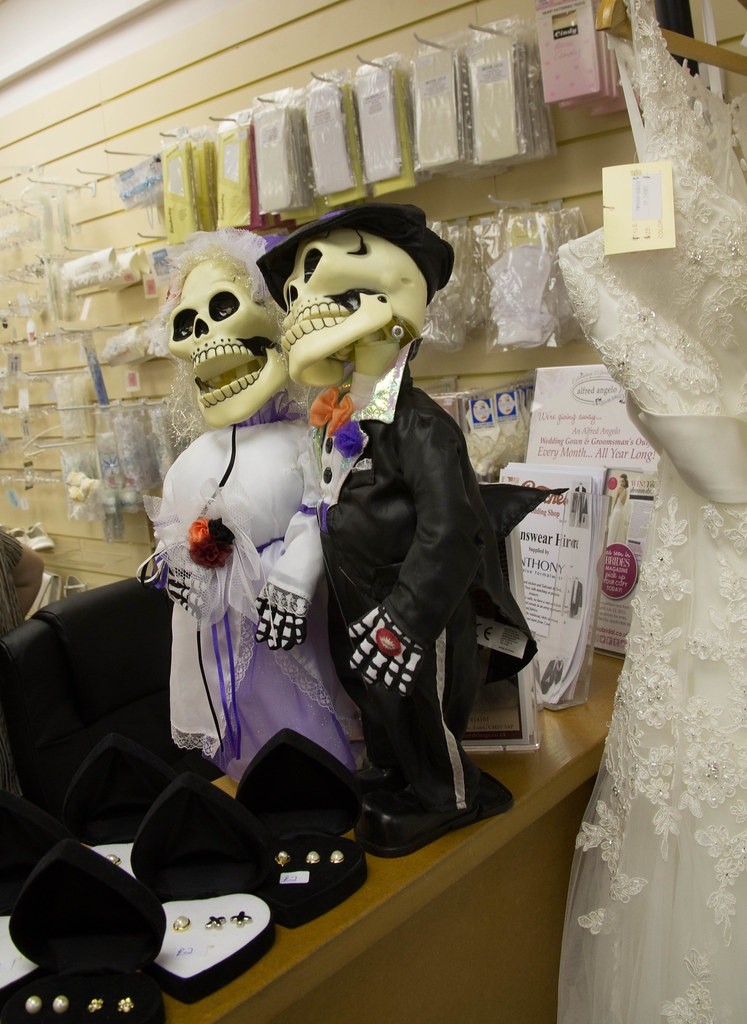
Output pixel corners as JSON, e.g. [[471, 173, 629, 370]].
[[0, 840, 167, 1024], [62, 733, 177, 880], [131, 771, 277, 1004], [0, 791, 76, 1010], [236, 727, 368, 929]]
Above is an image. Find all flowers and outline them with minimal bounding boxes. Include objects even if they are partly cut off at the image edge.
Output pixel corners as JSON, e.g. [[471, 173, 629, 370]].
[[190, 519, 235, 570], [334, 419, 362, 459]]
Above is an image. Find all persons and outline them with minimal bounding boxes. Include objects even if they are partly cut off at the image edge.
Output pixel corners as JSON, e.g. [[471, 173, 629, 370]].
[[0, 530, 44, 637], [607, 474, 629, 547]]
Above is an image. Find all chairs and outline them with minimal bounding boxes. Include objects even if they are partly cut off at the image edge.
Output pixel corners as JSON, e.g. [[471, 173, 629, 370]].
[[0, 578, 227, 824]]
